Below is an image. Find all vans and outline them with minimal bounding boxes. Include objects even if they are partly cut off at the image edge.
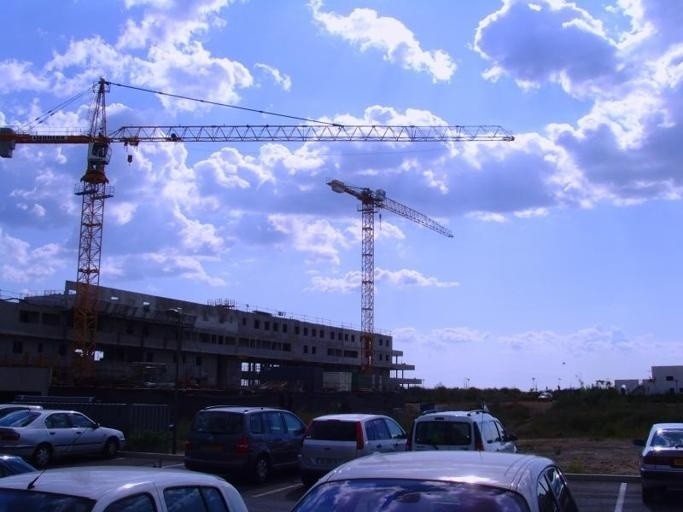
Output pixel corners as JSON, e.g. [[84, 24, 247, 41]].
[[183, 404, 308, 483], [411, 408, 518, 454], [296, 412, 409, 487]]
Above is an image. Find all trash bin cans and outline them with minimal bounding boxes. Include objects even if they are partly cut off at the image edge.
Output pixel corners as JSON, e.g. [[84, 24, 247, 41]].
[[167, 424, 177, 454]]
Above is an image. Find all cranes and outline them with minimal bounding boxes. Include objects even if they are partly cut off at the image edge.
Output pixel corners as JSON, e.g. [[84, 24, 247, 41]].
[[0, 75, 516, 387], [326, 178, 455, 393]]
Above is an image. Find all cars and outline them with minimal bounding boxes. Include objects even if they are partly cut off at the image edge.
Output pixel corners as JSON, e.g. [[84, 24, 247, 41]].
[[288, 449, 581, 512], [0, 402, 250, 512], [538, 392, 554, 403], [632, 422, 683, 505]]
